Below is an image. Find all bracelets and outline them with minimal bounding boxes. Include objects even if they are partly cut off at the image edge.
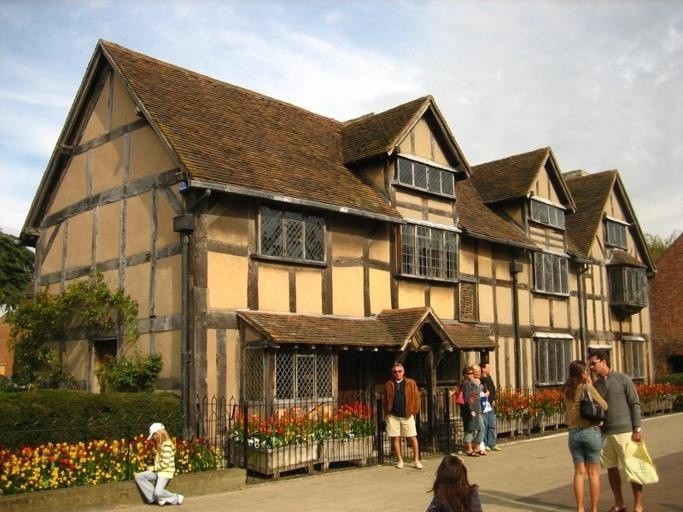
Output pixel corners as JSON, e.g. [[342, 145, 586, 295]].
[[632, 426, 642, 431]]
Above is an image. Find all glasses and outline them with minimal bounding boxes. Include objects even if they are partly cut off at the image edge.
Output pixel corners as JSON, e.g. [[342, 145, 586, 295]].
[[589, 359, 600, 364], [394, 371, 401, 373]]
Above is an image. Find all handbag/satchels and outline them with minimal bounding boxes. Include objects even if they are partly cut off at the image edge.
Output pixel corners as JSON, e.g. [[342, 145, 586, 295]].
[[455, 392, 474, 405], [580, 385, 606, 420], [625, 440, 659, 485]]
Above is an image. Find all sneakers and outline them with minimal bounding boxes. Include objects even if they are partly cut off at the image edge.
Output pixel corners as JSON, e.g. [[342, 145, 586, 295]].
[[485, 446, 501, 451], [397, 461, 403, 468], [415, 462, 422, 468]]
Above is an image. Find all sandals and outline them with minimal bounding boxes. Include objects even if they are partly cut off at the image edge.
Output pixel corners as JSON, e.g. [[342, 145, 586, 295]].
[[467, 450, 486, 456]]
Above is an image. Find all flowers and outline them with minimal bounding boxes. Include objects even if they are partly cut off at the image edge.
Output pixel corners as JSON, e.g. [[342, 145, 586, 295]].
[[495, 384, 566, 425], [227, 400, 379, 452], [635, 382, 683, 404]]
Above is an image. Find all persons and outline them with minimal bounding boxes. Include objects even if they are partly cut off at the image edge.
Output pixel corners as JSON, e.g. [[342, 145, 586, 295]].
[[585, 350, 646, 512], [456, 367, 487, 457], [426, 454, 483, 511], [478, 361, 502, 451], [560, 359, 609, 512], [464, 363, 489, 454], [381, 362, 422, 469], [132, 421, 184, 505]]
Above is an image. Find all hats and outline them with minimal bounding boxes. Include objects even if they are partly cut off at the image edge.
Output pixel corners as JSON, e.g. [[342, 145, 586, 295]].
[[147, 423, 164, 440]]
